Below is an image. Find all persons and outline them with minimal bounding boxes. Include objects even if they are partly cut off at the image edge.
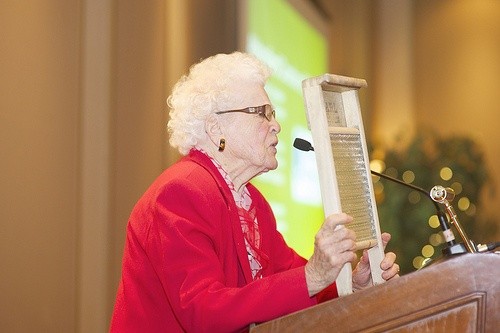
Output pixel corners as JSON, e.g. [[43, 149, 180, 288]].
[[105, 50, 402, 333]]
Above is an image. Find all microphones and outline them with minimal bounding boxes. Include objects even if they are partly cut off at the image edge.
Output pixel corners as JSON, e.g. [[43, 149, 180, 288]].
[[291, 137, 475, 258]]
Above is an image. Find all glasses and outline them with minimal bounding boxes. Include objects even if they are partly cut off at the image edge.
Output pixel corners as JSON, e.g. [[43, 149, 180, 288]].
[[216, 102, 276, 123]]
